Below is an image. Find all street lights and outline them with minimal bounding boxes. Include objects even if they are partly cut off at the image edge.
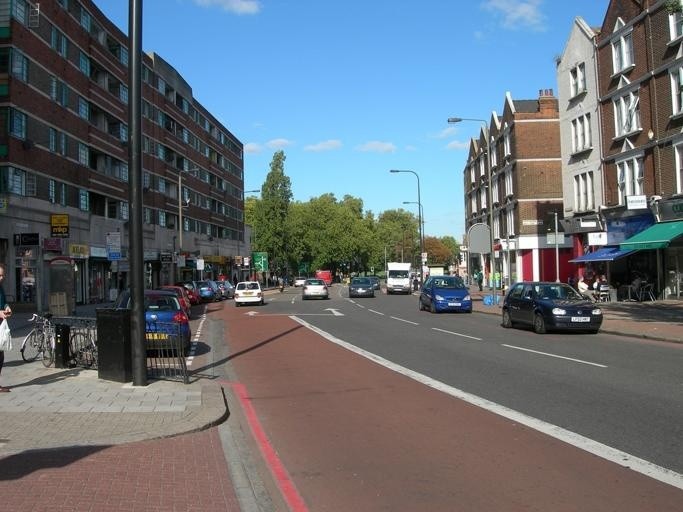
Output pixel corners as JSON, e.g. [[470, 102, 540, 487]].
[[237, 190, 260, 282], [448, 118, 497, 305], [179, 168, 199, 253], [340, 259, 350, 278], [390, 169, 425, 289]]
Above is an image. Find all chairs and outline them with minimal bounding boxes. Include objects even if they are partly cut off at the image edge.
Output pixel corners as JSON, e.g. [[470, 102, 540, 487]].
[[567, 281, 656, 302], [528, 290, 538, 299], [551, 289, 564, 299]]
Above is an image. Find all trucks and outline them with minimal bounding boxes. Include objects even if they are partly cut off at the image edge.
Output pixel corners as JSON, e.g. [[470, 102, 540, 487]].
[[315, 270, 334, 286], [387, 262, 412, 295]]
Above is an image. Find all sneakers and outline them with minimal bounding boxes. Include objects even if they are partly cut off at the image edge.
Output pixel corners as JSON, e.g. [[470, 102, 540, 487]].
[[0, 386, 9, 393]]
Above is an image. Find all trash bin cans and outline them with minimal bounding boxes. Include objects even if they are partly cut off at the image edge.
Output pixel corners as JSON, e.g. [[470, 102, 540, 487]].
[[95, 308, 132, 383]]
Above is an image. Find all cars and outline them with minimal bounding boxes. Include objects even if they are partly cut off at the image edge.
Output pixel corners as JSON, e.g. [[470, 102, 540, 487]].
[[114, 281, 263, 357], [293, 276, 329, 300], [419, 275, 472, 314], [348, 275, 380, 298], [503, 281, 603, 334]]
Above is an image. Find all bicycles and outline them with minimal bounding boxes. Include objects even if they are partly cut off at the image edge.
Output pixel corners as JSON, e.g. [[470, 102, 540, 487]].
[[20, 313, 96, 367]]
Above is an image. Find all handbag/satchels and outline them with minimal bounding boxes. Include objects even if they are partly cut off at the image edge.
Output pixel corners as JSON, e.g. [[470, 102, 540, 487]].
[[0, 319, 12, 352]]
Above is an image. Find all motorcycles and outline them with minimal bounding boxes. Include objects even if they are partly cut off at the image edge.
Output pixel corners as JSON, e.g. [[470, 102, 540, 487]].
[[279, 285, 284, 292]]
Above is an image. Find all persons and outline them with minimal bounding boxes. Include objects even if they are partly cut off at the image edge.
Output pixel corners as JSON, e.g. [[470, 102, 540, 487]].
[[475, 268, 484, 291], [577, 271, 643, 302], [0, 262, 12, 379]]
